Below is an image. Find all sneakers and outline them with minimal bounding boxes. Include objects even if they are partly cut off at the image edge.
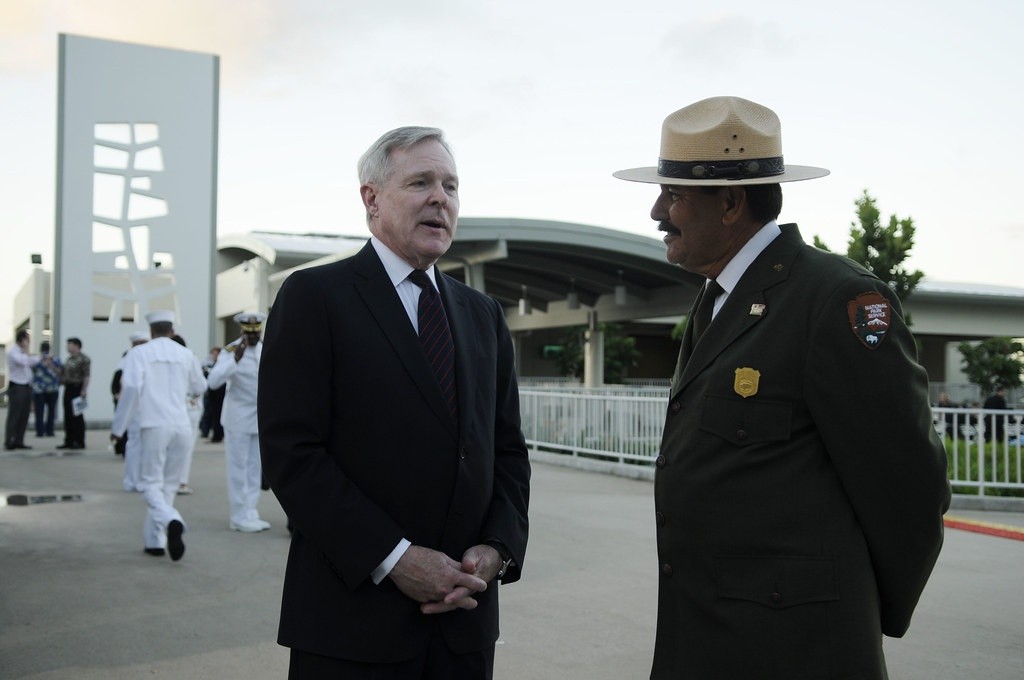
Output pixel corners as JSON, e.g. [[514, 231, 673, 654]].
[[254, 519, 270, 530], [229, 520, 262, 533]]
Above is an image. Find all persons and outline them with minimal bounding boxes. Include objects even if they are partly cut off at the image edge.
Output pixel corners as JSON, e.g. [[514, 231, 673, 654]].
[[110, 308, 208, 562], [259, 126, 532, 680], [613, 96, 952, 680], [57, 338, 90, 448], [5, 329, 32, 450], [208, 312, 271, 532], [31, 343, 60, 437], [200, 348, 225, 442]]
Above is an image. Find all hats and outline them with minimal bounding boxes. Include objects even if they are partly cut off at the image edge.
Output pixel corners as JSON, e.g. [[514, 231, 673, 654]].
[[129, 332, 150, 343], [145, 310, 174, 324], [612, 97, 830, 185], [233, 311, 267, 332]]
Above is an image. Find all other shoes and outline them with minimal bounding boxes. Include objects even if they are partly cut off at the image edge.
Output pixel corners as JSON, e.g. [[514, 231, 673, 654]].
[[167, 520, 185, 561], [144, 547, 165, 556], [178, 485, 193, 494]]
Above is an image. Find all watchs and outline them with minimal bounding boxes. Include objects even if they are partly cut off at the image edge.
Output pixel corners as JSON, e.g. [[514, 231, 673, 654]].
[[485, 540, 517, 580]]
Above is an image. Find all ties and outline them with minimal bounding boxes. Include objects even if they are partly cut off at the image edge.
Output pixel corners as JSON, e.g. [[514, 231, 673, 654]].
[[407, 269, 457, 417], [691, 281, 726, 348]]
